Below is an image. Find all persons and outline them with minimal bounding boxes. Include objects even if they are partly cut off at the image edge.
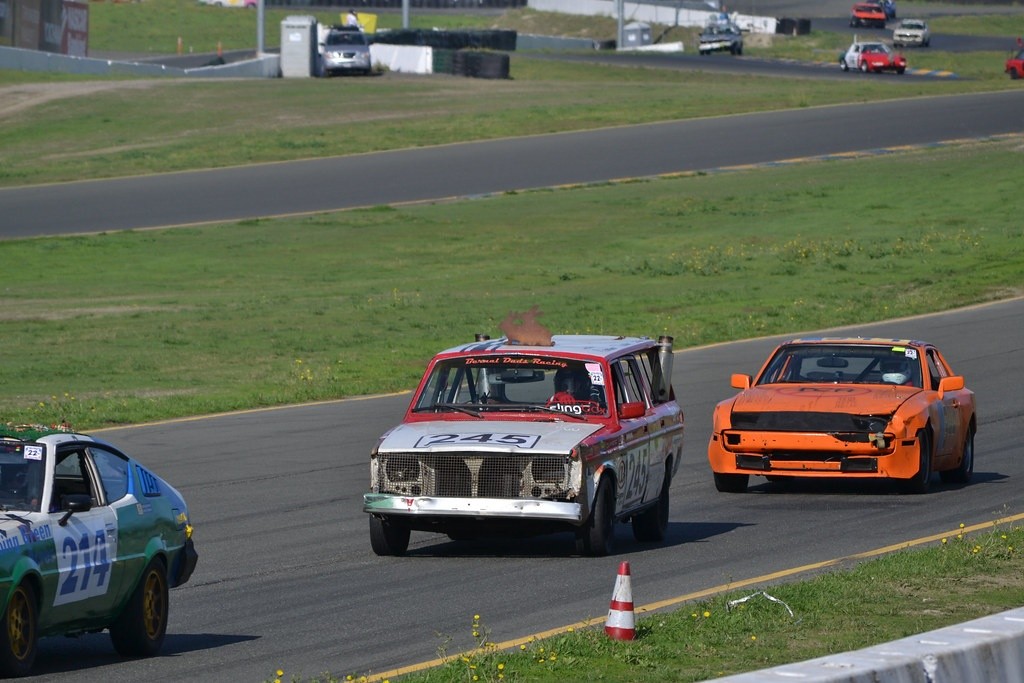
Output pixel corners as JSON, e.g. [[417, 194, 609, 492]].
[[345, 8, 361, 30], [877, 357, 914, 386], [710, 2, 731, 35], [2, 463, 46, 510], [549, 369, 603, 413]]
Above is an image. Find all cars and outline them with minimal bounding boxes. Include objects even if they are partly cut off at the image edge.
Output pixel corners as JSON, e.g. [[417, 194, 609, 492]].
[[891, 19, 930, 48], [317, 29, 373, 77], [698, 22, 743, 57], [1004, 45, 1024, 80], [838, 41, 907, 74], [849, 2, 886, 30]]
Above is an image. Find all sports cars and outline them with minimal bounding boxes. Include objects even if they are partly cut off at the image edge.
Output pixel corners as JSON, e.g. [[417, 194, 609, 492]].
[[1, 425, 197, 683], [363, 328, 687, 556], [708, 335, 976, 492]]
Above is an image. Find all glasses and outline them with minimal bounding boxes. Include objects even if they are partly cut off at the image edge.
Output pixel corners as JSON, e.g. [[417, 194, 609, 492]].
[[559, 383, 580, 391]]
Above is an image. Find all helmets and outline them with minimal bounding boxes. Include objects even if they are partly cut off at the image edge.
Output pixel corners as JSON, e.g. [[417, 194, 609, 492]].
[[554, 362, 591, 400], [880, 357, 912, 384], [0, 453, 28, 497]]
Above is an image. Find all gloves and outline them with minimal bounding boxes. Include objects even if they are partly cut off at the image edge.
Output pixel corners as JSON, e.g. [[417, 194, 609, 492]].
[[550, 392, 575, 404]]
